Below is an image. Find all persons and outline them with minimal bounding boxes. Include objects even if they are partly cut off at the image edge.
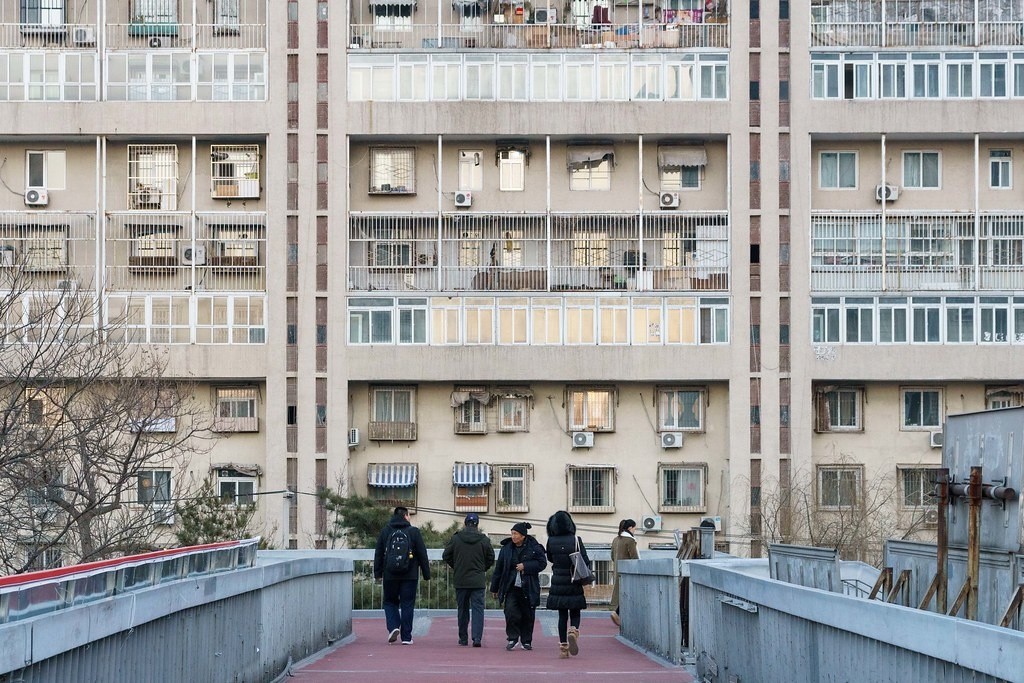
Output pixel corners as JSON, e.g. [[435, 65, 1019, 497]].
[[610, 519, 640, 626], [442, 513, 495, 647], [490, 522, 548, 652], [374, 507, 431, 645], [546, 510, 589, 659]]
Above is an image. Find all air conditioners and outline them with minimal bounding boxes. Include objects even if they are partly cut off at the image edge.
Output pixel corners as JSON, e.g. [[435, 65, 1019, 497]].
[[148, 37, 171, 48], [659, 192, 679, 207], [56, 280, 76, 295], [73, 27, 93, 46], [23, 428, 45, 445], [661, 432, 682, 448], [534, 8, 556, 24], [643, 515, 662, 531], [573, 431, 593, 448], [34, 507, 58, 523], [924, 508, 938, 525], [416, 252, 434, 268], [351, 428, 360, 444], [137, 186, 156, 205], [154, 510, 175, 525], [930, 431, 942, 448], [699, 515, 722, 531], [23, 187, 49, 205], [182, 244, 206, 265], [875, 184, 898, 200], [0, 250, 15, 265], [454, 191, 472, 207]]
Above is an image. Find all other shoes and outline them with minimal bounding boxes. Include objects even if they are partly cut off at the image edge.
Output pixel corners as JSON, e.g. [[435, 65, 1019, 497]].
[[388, 628, 399, 642], [474, 643, 481, 647], [402, 639, 413, 645], [459, 640, 467, 645], [611, 611, 620, 626]]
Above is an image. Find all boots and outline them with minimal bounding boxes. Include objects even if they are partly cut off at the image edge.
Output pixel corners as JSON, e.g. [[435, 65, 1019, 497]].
[[558, 642, 569, 659], [567, 625, 580, 656]]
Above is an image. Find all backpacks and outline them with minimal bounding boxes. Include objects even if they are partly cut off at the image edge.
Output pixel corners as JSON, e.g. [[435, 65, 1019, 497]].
[[383, 524, 413, 572]]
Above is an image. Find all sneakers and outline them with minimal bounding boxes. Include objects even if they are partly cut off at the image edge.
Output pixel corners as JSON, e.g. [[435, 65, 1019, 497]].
[[522, 644, 531, 650], [507, 641, 519, 649]]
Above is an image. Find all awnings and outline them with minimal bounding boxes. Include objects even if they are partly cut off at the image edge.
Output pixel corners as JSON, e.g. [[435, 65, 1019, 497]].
[[453, 463, 491, 487], [368, 464, 418, 488]]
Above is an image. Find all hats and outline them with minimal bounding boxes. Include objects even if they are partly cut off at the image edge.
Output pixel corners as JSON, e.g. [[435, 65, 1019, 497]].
[[465, 514, 479, 522], [511, 522, 532, 536]]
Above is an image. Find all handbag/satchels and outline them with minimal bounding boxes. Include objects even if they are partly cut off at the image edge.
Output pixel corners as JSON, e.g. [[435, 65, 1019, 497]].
[[569, 536, 595, 586]]
